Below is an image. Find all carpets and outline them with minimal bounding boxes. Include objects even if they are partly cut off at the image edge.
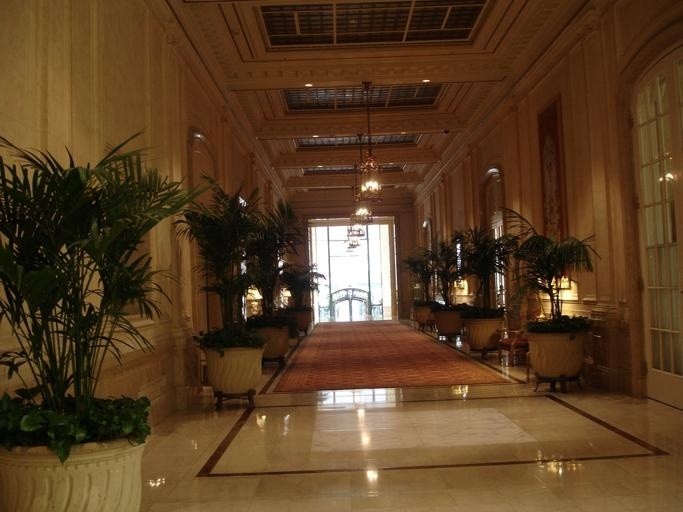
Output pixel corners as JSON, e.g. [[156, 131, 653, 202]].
[[273, 321, 512, 392]]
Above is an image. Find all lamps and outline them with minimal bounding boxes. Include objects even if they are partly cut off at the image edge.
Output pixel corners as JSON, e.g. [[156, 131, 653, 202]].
[[346, 81, 381, 248]]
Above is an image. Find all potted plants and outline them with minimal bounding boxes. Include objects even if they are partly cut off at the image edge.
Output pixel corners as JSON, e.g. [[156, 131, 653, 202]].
[[402, 253, 440, 332], [425, 239, 470, 344], [493, 205, 603, 393], [246, 195, 304, 370], [281, 259, 327, 337], [0, 131, 216, 512], [176, 171, 284, 410], [455, 225, 518, 360]]
